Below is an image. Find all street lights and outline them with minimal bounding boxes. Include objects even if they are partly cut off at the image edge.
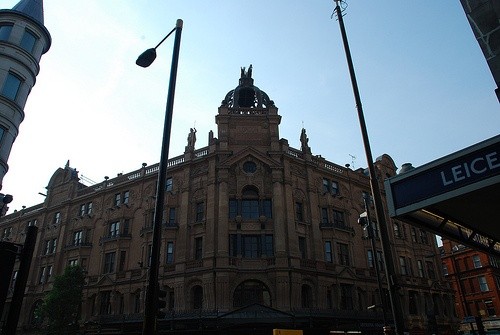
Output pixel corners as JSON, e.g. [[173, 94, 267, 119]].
[[356, 198, 393, 335], [135, 18, 184, 335]]
